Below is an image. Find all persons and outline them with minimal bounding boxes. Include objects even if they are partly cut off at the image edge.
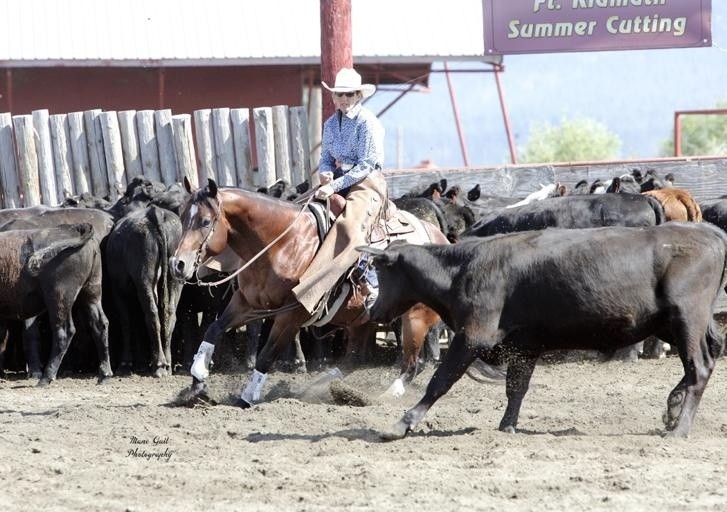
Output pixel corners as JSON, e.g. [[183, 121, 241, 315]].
[[316, 90, 385, 313]]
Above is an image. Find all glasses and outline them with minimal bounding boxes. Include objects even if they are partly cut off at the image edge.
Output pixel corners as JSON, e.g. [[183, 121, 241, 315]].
[[336, 92, 354, 97]]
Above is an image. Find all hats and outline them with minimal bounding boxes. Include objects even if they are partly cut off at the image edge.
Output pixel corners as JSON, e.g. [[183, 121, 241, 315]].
[[321, 67, 376, 97]]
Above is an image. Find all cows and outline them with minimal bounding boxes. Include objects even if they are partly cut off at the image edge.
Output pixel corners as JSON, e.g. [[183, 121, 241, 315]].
[[0, 174, 190, 389], [352, 167, 727, 443]]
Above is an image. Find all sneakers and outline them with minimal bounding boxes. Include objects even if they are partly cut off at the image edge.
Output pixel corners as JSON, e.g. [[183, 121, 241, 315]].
[[365, 288, 378, 310]]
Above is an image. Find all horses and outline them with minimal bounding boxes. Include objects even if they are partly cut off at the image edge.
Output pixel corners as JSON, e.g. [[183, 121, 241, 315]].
[[165, 175, 456, 413]]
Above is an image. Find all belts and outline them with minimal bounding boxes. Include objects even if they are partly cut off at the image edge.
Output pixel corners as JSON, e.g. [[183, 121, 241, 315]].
[[375, 165, 381, 172]]
